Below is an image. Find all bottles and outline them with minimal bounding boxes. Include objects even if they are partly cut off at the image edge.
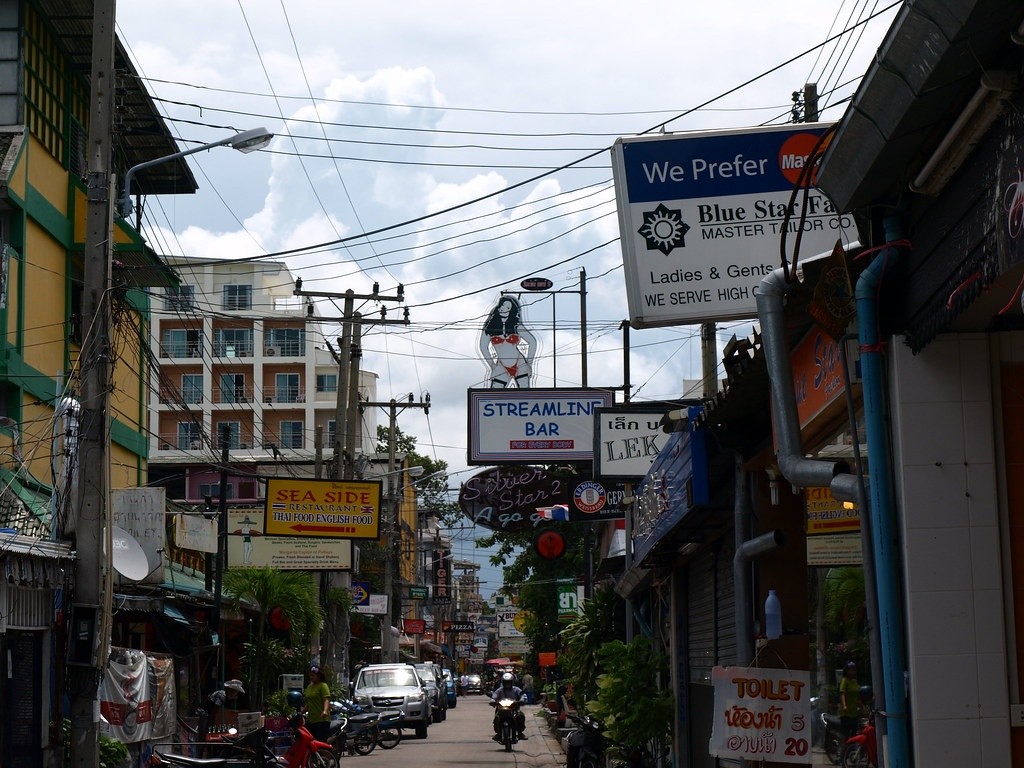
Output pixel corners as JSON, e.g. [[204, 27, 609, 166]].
[[765, 590, 781, 640]]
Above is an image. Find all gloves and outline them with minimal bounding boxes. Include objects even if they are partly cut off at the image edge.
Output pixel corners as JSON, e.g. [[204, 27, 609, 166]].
[[489, 699, 496, 708], [519, 699, 524, 705]]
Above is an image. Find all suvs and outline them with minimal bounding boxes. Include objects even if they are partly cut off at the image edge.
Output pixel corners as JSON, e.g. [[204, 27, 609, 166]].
[[349, 662, 434, 739], [412, 660, 459, 722]]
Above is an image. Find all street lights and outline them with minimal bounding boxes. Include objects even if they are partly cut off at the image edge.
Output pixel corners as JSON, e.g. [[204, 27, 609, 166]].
[[413, 552, 456, 665], [331, 465, 426, 688], [60, 124, 275, 768], [378, 470, 451, 665]]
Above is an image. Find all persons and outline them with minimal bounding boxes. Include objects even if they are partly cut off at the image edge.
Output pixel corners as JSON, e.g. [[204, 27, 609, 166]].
[[461, 672, 469, 698], [196, 679, 245, 760], [352, 664, 362, 683], [489, 673, 529, 740], [301, 666, 331, 749], [522, 672, 533, 704]]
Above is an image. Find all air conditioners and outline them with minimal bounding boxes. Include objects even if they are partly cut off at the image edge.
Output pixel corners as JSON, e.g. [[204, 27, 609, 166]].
[[239, 351, 252, 357], [161, 352, 175, 358], [160, 398, 175, 404], [264, 396, 278, 403], [239, 442, 253, 449], [262, 441, 279, 449], [266, 346, 281, 357], [161, 442, 176, 450], [238, 397, 253, 403]]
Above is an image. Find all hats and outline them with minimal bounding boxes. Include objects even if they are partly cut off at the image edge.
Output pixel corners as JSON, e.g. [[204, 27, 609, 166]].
[[462, 672, 467, 675], [224, 679, 245, 693]]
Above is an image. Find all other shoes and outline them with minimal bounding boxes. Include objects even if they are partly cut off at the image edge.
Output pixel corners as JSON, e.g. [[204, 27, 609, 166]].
[[492, 734, 502, 741], [518, 733, 528, 740]]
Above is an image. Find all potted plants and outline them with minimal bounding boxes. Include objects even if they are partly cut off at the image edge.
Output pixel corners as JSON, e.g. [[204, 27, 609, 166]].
[[542, 684, 558, 712]]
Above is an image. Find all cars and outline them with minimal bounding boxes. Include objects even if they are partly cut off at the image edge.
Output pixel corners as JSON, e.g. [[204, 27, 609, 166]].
[[466, 674, 482, 689]]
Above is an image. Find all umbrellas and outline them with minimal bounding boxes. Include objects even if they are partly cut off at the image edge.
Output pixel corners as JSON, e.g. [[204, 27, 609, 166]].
[[485, 659, 507, 667]]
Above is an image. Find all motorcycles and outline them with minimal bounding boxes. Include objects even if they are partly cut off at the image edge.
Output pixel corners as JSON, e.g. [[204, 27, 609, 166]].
[[486, 690, 527, 753], [178, 680, 407, 756], [148, 691, 341, 768]]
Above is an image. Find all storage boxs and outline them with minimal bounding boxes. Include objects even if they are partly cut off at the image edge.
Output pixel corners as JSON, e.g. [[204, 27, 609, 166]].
[[269, 731, 291, 747], [274, 746, 291, 755], [205, 733, 238, 758], [238, 712, 261, 736]]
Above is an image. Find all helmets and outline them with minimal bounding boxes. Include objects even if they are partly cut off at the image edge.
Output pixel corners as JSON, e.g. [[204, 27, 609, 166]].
[[502, 673, 514, 688], [285, 691, 304, 707]]
